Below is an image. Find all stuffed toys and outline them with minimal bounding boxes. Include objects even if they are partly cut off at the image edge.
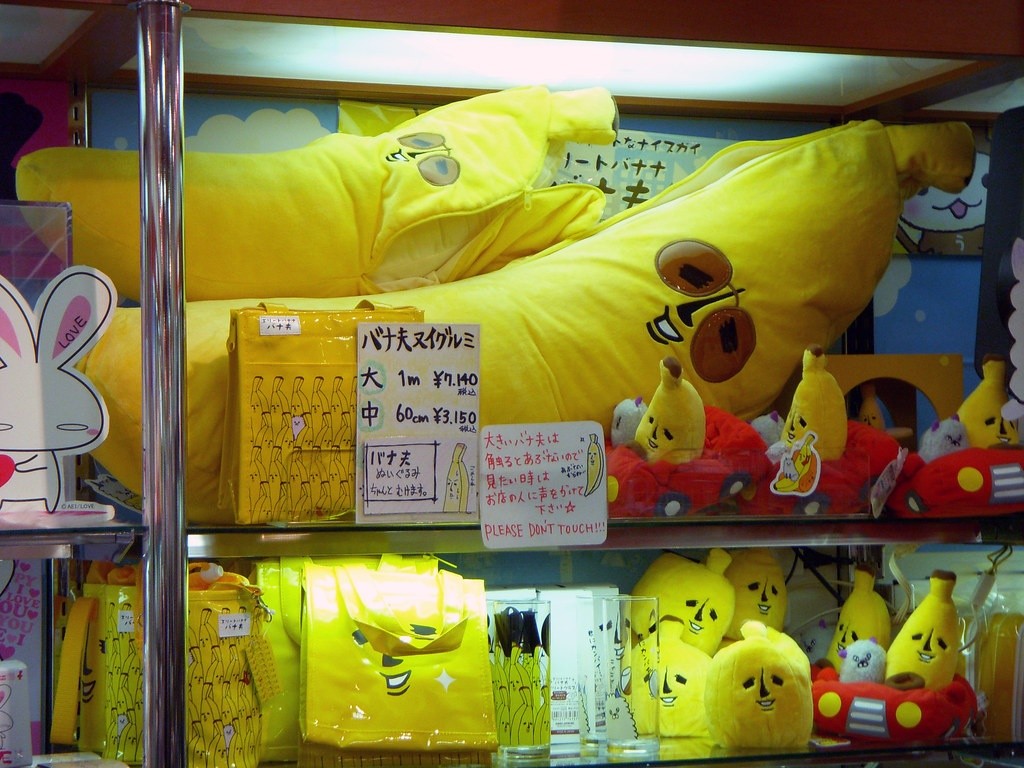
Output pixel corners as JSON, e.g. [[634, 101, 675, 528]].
[[618, 546, 981, 748], [13, 81, 1024, 516]]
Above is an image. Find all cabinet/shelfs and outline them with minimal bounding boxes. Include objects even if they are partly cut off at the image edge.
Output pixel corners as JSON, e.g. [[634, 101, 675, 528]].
[[1, 0, 1024, 766]]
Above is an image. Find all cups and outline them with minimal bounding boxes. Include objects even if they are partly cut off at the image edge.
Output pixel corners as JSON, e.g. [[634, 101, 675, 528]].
[[487, 598, 538, 760], [601, 595, 660, 755], [574, 594, 632, 758], [493, 600, 551, 768]]
